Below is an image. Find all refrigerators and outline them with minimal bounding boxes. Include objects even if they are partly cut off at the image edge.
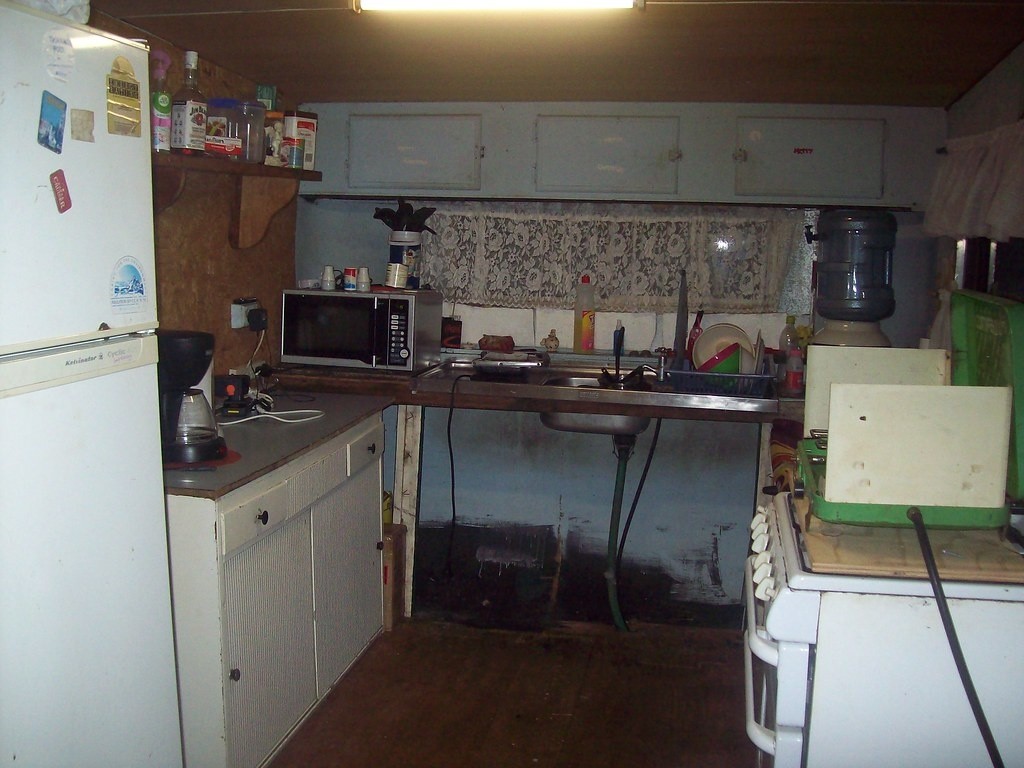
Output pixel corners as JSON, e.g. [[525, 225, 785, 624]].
[[0, 0, 191, 768]]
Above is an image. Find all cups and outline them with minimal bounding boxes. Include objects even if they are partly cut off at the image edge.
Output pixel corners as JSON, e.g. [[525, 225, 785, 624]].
[[283, 137, 304, 168], [390, 231, 422, 289]]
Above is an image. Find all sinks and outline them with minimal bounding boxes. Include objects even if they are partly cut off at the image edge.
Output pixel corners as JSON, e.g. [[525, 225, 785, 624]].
[[539, 376, 651, 436]]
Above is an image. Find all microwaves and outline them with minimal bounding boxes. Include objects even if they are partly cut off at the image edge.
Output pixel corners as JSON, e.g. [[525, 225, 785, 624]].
[[281, 284, 443, 372]]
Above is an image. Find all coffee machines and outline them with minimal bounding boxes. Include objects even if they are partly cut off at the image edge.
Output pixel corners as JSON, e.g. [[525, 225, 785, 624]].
[[155, 327, 226, 461]]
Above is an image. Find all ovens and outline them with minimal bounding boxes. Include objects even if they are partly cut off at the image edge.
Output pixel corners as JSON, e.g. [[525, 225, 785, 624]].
[[742, 549, 1024, 768]]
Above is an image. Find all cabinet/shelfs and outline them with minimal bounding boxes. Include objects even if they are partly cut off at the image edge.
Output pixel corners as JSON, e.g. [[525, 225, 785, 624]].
[[166, 410, 386, 768]]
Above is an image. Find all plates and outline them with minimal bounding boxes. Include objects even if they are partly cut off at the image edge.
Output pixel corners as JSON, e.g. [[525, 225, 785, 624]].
[[755, 329, 766, 375]]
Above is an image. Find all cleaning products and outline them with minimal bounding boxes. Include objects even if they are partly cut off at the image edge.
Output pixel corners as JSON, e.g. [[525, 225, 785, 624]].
[[573, 273, 595, 355]]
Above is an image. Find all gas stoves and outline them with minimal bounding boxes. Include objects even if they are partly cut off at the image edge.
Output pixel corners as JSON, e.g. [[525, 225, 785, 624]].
[[751, 492, 1023, 644]]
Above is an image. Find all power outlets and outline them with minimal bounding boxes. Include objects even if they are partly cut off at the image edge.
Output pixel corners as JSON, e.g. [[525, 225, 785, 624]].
[[229, 302, 257, 331]]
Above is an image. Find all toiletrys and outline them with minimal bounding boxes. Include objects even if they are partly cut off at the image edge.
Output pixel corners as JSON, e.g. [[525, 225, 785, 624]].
[[613, 320, 625, 357]]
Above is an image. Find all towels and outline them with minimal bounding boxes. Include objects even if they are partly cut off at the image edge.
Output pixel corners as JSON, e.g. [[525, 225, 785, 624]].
[[768, 418, 803, 474]]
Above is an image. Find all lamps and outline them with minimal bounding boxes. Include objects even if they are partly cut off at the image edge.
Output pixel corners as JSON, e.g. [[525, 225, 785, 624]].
[[346, 0, 650, 12]]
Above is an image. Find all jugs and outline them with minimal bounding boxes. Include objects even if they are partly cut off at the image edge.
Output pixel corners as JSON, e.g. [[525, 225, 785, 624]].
[[176, 389, 218, 443]]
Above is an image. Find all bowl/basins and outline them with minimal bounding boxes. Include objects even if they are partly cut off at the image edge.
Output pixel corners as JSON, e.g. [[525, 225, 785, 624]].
[[692, 323, 755, 375]]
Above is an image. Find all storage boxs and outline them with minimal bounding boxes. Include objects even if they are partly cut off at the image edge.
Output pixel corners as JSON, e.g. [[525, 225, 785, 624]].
[[663, 353, 777, 397], [383, 524, 406, 632]]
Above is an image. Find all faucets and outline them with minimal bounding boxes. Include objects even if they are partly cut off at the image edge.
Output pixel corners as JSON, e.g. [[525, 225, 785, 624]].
[[601, 326, 643, 384], [640, 346, 672, 382]]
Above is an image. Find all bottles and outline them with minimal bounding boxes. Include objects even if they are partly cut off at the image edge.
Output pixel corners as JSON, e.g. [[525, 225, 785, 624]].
[[320, 264, 370, 292], [764, 316, 804, 398], [265, 112, 284, 167], [814, 208, 897, 321], [150, 51, 170, 155], [171, 50, 208, 153], [573, 274, 595, 354]]
